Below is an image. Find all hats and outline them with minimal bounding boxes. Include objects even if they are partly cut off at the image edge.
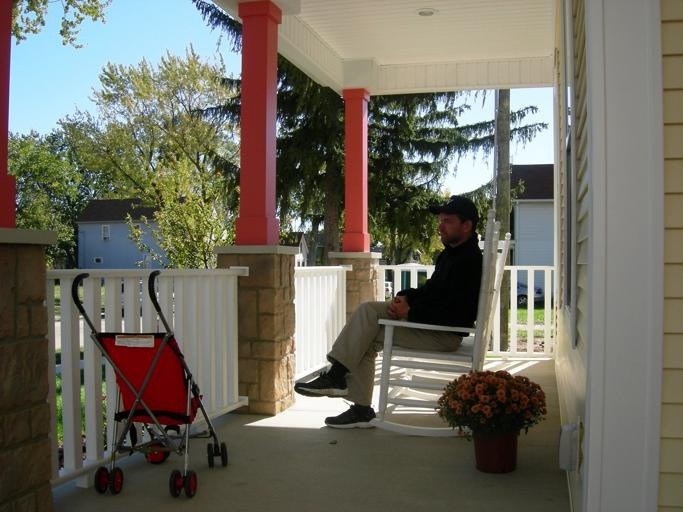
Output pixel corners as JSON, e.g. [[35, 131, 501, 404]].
[[426, 194, 480, 221]]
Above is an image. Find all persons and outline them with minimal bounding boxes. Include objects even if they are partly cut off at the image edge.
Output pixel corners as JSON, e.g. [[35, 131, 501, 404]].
[[292, 193, 484, 429]]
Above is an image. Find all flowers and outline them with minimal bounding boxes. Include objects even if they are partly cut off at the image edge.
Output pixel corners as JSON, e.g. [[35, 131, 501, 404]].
[[434, 370, 548, 443]]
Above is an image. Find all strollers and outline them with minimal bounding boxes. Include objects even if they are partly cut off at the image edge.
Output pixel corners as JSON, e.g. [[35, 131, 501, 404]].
[[72, 269, 228, 498]]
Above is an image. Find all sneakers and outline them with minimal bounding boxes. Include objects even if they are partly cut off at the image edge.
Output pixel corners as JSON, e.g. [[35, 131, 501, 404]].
[[323, 404, 377, 430], [293, 370, 350, 399]]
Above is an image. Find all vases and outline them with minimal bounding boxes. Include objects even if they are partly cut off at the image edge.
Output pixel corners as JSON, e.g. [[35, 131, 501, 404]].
[[474, 433, 519, 473]]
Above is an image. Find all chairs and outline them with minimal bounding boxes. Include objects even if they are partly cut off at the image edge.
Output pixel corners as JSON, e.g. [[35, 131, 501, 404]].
[[369, 232, 511, 438]]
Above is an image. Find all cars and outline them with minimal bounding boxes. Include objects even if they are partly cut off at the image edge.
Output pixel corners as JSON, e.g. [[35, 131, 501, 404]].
[[518, 281, 543, 306], [122, 280, 175, 317], [385, 281, 393, 298]]
